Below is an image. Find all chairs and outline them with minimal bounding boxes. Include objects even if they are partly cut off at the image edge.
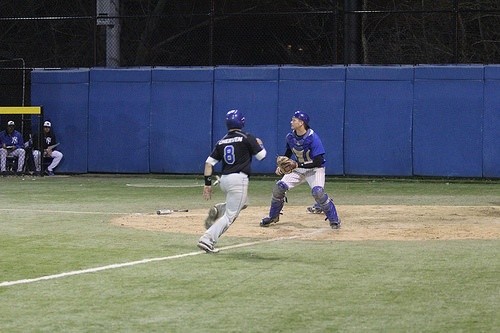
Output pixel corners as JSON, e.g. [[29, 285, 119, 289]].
[[30, 133, 53, 174]]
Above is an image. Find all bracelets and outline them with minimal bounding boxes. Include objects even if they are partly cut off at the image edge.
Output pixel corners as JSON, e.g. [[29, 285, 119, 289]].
[[204, 175, 211, 186]]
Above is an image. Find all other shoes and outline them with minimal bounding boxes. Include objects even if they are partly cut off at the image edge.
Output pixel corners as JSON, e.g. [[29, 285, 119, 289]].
[[260, 214, 280, 226], [197, 241, 219, 253], [35, 171, 40, 177], [16, 172, 22, 176], [330, 221, 340, 230], [0, 171, 7, 177], [205, 205, 218, 230], [45, 168, 54, 176]]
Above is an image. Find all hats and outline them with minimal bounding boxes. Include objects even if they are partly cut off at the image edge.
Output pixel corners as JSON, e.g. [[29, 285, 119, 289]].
[[8, 121, 15, 126], [43, 121, 51, 127]]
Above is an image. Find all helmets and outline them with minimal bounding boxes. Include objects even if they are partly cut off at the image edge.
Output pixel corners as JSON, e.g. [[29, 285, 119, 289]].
[[294, 111, 311, 128], [224, 109, 246, 129]]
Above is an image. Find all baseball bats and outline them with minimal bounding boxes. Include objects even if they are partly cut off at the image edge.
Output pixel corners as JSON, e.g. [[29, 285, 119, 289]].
[[156, 209, 188, 216]]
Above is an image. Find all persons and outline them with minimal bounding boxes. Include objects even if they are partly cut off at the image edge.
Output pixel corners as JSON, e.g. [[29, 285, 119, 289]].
[[260, 111, 341, 229], [0, 120, 25, 171], [197, 110, 266, 253], [32, 121, 63, 176]]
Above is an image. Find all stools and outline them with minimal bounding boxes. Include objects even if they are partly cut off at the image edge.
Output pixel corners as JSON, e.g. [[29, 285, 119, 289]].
[[6, 155, 19, 172]]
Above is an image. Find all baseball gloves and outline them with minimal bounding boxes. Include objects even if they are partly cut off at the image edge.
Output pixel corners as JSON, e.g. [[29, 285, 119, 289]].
[[277, 156, 297, 175]]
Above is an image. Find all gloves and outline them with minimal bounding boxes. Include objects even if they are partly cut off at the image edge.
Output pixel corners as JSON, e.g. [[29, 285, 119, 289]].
[[281, 159, 297, 171]]
